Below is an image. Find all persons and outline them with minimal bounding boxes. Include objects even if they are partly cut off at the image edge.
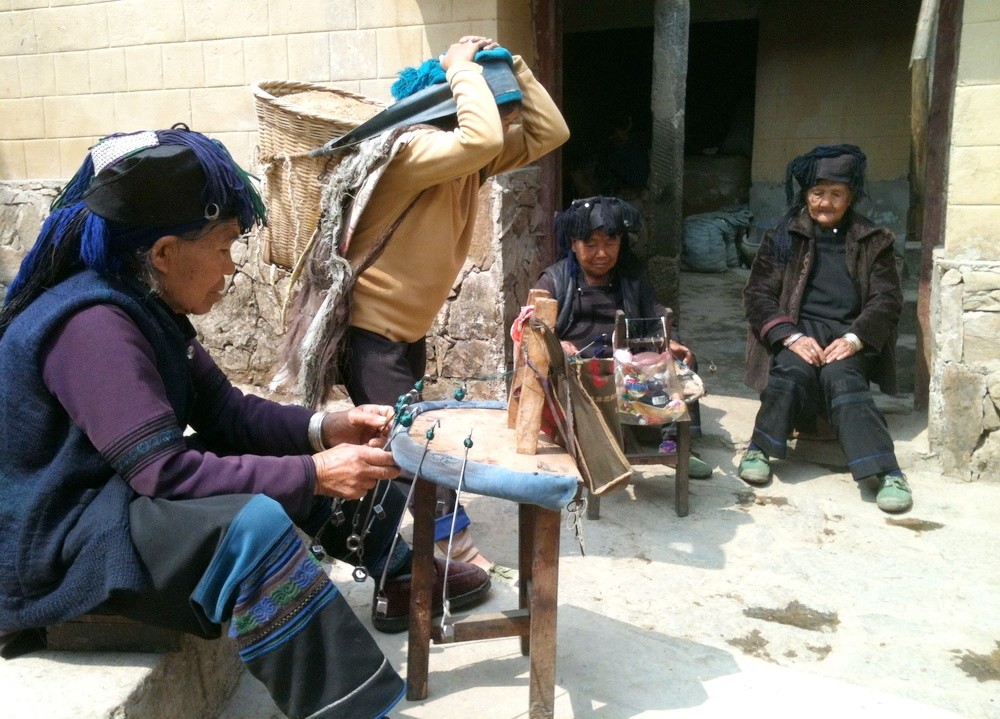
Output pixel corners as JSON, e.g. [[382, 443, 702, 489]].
[[0, 126, 494, 719], [336, 34, 570, 595], [536, 197, 714, 477], [737, 143, 913, 512]]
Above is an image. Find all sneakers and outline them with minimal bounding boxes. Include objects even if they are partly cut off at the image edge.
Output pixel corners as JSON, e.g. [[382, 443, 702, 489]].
[[737, 449, 770, 483], [658, 444, 712, 478], [876, 473, 913, 511], [371, 553, 491, 634], [486, 561, 520, 586]]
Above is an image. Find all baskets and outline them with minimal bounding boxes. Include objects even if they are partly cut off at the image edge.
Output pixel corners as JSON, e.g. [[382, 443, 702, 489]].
[[251, 77, 391, 274]]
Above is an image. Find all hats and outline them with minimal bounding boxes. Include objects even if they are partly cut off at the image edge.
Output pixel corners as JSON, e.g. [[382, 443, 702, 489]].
[[553, 194, 642, 242], [785, 143, 867, 217]]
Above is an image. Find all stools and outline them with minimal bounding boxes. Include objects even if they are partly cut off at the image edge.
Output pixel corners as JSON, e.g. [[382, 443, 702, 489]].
[[396, 288, 584, 719], [585, 359, 692, 521]]
